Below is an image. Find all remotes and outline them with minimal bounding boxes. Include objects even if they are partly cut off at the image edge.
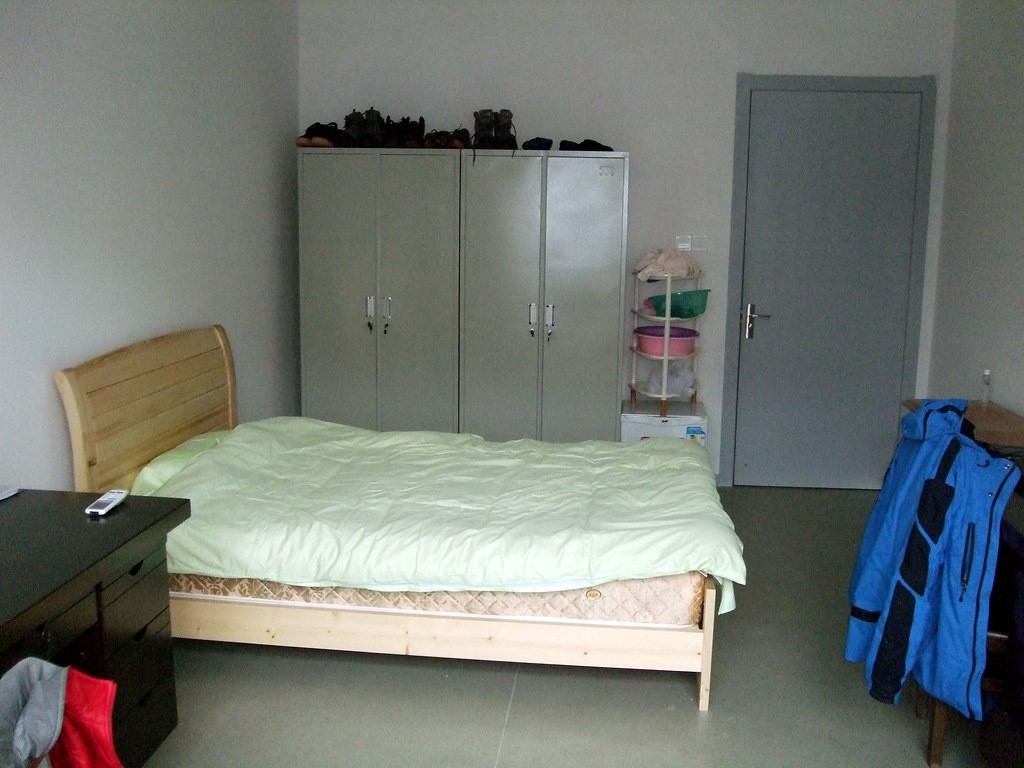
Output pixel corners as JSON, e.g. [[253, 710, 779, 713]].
[[85, 490, 129, 516]]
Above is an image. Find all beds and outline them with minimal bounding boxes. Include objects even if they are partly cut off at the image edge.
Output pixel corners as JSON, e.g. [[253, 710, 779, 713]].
[[52, 325, 750, 717]]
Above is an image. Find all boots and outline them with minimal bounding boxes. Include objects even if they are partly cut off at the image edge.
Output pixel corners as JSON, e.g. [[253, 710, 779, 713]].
[[470, 109, 518, 166]]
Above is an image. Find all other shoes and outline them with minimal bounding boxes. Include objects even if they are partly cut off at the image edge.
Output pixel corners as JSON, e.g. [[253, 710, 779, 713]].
[[559, 139, 613, 151], [522, 137, 553, 150], [297, 107, 471, 147]]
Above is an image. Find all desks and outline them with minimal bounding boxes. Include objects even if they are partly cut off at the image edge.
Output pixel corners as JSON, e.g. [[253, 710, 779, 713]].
[[0, 489, 192, 768], [899, 396, 1024, 554]]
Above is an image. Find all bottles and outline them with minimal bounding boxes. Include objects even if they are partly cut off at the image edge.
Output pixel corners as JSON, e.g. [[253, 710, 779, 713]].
[[977, 370, 990, 407]]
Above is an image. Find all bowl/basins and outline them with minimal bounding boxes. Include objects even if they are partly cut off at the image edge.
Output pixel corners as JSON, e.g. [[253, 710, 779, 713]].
[[632, 325, 700, 357], [648, 289, 711, 319]]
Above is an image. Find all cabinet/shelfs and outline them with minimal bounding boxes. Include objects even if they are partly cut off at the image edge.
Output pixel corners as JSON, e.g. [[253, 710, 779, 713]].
[[295, 143, 631, 453], [629, 262, 704, 418]]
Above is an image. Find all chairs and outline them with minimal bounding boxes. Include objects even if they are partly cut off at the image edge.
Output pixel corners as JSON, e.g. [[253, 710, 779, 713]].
[[841, 398, 1019, 768]]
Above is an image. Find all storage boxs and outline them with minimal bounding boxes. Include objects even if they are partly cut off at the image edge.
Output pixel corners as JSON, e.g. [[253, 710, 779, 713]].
[[621, 398, 709, 452]]
